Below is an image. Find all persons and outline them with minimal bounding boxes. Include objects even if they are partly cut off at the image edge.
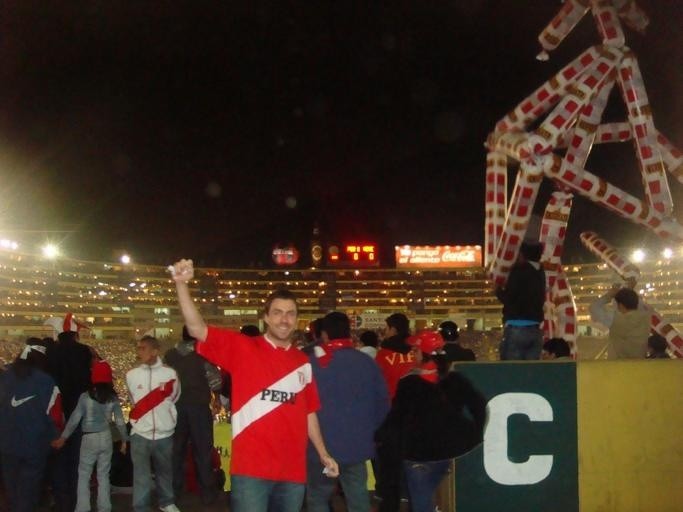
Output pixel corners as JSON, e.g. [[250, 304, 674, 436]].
[[645, 333, 670, 359], [494, 242, 545, 360], [1, 257, 494, 511], [590, 276, 652, 358], [541, 338, 571, 362]]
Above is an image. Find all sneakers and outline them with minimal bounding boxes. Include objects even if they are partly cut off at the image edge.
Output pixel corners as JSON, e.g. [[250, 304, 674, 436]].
[[159, 503, 182, 512]]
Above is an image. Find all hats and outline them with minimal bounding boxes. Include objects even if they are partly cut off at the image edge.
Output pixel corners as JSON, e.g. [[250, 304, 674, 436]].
[[42, 314, 91, 338], [90, 359, 113, 385], [406, 330, 448, 355]]
